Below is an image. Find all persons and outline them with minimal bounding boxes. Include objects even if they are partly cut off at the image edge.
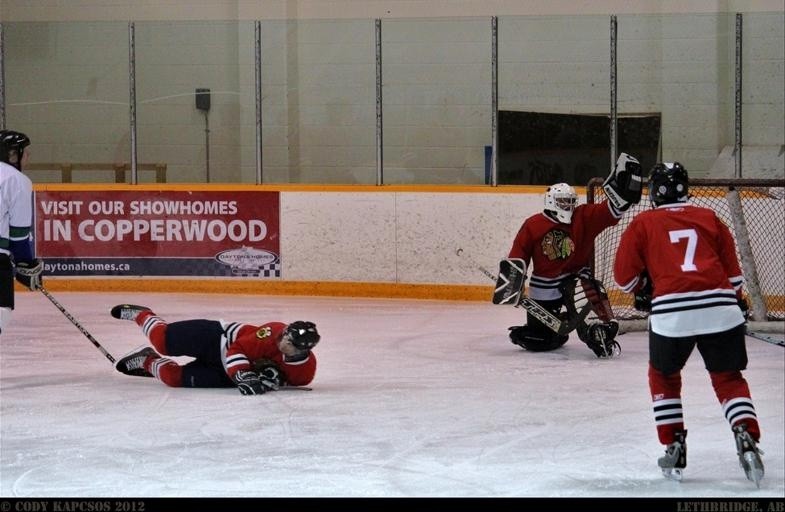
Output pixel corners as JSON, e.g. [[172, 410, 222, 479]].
[[492, 181, 631, 357], [109, 303, 321, 396], [1, 129, 45, 340], [612, 160, 766, 484]]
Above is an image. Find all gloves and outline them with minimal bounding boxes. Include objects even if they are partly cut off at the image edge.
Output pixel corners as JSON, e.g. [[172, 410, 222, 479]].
[[234, 370, 265, 394], [258, 367, 280, 391], [635, 279, 652, 312], [15, 261, 45, 291]]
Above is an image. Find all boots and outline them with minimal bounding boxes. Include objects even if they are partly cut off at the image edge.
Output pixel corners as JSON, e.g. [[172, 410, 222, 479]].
[[732, 422, 764, 481], [110, 304, 150, 321], [657, 430, 688, 469], [116, 347, 156, 373]]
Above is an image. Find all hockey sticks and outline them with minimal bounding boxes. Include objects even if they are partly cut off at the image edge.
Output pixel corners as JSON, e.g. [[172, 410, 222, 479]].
[[457, 249, 592, 336]]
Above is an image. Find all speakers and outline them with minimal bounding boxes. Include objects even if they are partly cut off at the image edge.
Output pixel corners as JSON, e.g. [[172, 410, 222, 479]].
[[195, 88, 210, 109]]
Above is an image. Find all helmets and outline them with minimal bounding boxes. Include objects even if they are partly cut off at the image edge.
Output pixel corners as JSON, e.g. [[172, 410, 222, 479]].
[[284, 321, 320, 350], [0, 129, 31, 151], [648, 161, 688, 209], [544, 183, 578, 225]]
